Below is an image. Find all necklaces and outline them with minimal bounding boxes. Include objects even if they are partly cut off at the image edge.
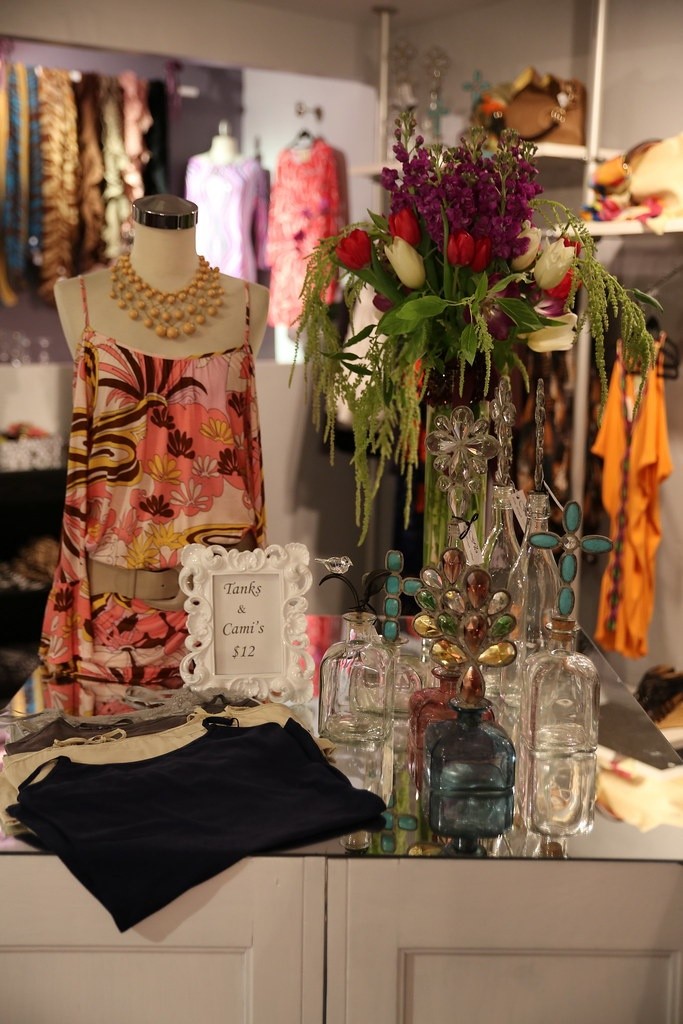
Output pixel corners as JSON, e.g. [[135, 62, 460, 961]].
[[110, 251, 224, 337]]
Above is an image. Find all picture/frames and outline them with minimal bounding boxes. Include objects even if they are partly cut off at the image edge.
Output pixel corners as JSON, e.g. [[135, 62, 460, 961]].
[[179, 543, 316, 706]]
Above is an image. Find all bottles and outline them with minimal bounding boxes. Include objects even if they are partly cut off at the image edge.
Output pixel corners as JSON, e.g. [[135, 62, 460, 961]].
[[318, 486, 600, 840]]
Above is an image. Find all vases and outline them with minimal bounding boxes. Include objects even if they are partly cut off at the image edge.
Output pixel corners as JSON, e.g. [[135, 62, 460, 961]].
[[424, 398, 490, 591]]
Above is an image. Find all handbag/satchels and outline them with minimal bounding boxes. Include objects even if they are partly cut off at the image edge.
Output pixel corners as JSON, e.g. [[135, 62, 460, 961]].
[[579, 139, 663, 220], [464, 67, 588, 148]]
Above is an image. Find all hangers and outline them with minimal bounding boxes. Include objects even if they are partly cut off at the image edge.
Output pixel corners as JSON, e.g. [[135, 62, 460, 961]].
[[287, 112, 321, 155], [597, 295, 681, 383]]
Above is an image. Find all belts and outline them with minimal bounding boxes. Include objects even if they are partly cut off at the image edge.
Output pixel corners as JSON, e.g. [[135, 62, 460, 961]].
[[88, 530, 258, 613]]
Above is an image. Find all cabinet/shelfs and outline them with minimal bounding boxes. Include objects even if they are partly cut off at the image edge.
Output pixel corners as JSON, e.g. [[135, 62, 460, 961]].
[[0, 613, 683, 1024]]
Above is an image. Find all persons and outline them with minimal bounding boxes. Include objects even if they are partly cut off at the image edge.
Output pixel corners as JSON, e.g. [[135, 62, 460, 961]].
[[53, 194, 269, 681], [185, 134, 271, 285]]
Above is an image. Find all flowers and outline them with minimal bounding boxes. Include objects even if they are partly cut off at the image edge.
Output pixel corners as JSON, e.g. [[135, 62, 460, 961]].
[[336, 92, 583, 342]]
